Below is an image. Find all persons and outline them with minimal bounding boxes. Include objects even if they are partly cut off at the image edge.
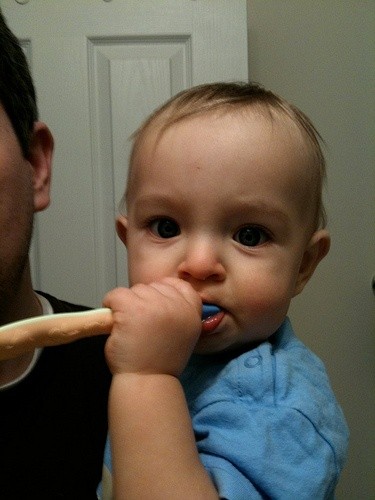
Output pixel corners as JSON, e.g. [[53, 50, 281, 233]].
[[0, 12, 112, 494], [102, 85, 351, 495]]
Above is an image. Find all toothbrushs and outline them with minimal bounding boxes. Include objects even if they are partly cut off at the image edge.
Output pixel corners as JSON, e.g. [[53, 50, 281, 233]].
[[1, 299, 223, 356]]
